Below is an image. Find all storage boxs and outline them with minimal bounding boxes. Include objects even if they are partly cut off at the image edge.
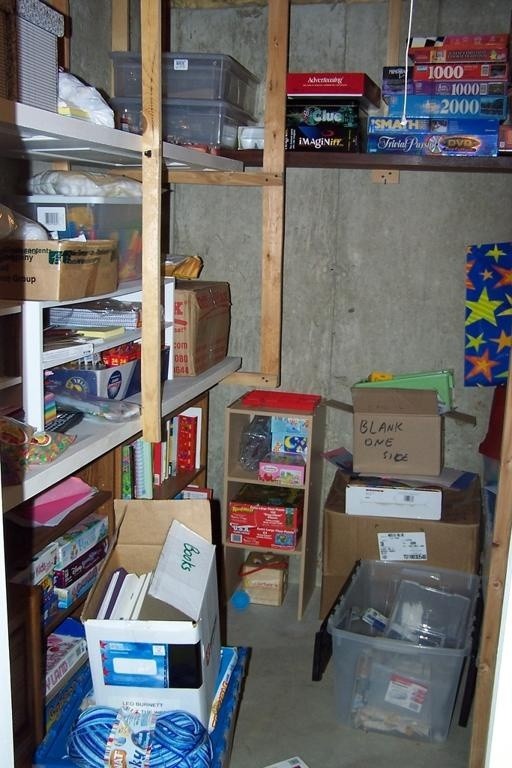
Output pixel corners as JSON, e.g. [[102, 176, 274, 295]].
[[356, 368, 457, 415], [323, 382, 477, 479], [313, 558, 487, 741], [242, 552, 291, 607], [230, 481, 302, 548], [107, 48, 264, 146], [176, 279, 234, 377], [297, 64, 508, 156], [78, 497, 223, 730], [258, 415, 307, 484], [322, 469, 484, 621], [2, 2, 66, 114], [2, 236, 122, 297]]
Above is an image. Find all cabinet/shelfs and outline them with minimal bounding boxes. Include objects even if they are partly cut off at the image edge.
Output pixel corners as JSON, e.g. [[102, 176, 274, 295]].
[[1, 279, 239, 768], [1, 0, 288, 437], [224, 389, 326, 618]]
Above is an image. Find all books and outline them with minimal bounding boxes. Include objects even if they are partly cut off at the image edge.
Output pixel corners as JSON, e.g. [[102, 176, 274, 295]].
[[3, 476, 109, 697], [42, 299, 142, 361], [95, 566, 193, 620], [284, 33, 512, 158], [122, 406, 213, 500]]
